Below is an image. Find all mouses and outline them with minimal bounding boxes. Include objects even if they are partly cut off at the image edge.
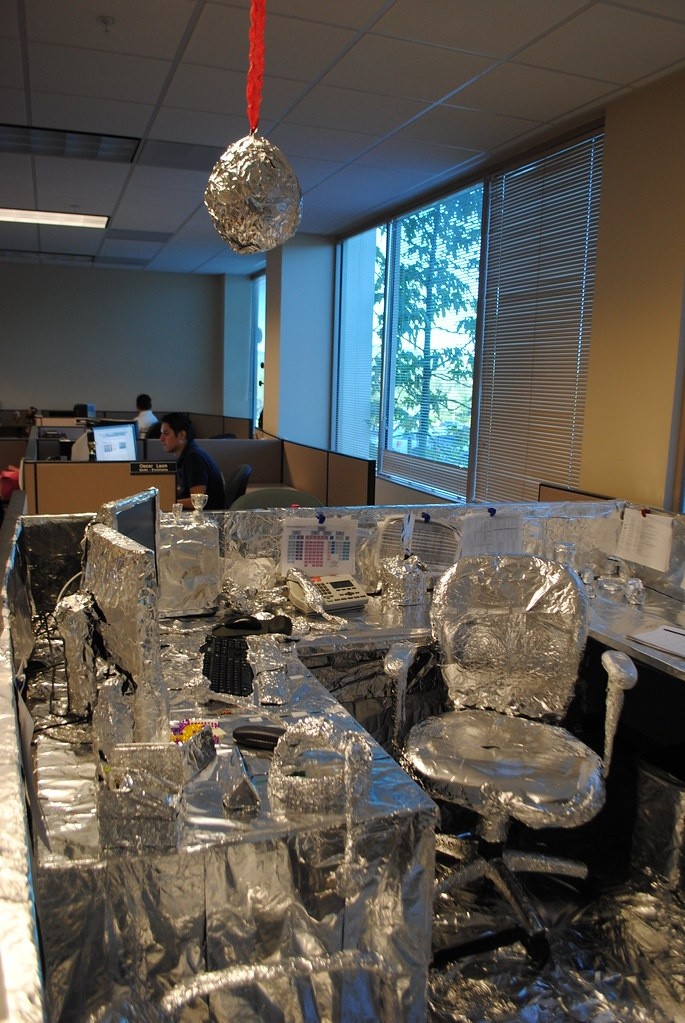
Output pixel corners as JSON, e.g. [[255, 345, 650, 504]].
[[225, 616, 263, 630]]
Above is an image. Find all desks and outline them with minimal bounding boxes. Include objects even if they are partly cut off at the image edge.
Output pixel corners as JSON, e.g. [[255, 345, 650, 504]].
[[28, 582, 685, 1023]]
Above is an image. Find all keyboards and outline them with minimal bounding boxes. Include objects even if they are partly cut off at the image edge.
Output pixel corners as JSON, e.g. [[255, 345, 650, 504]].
[[199, 636, 253, 697]]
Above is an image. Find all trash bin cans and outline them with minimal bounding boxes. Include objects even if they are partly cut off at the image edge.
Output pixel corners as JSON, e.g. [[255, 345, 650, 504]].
[[627, 737, 685, 887]]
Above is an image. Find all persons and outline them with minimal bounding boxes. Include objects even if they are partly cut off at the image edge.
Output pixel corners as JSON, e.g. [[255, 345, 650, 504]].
[[160, 413, 226, 509], [131, 394, 159, 439]]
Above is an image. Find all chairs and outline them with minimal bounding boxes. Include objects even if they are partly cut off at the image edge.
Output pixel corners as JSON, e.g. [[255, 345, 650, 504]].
[[220, 488, 326, 557], [226, 463, 252, 513], [383, 554, 637, 961]]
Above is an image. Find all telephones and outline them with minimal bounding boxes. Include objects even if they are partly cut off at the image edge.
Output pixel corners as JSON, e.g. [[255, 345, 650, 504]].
[[285, 566, 369, 617]]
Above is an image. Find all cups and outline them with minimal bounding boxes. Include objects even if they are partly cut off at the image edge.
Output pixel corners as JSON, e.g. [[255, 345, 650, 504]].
[[87, 403, 96, 417]]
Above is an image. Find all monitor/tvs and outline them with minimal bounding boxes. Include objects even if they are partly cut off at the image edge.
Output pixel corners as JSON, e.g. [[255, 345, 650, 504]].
[[86, 403, 96, 418], [85, 421, 140, 462], [100, 488, 163, 599]]
